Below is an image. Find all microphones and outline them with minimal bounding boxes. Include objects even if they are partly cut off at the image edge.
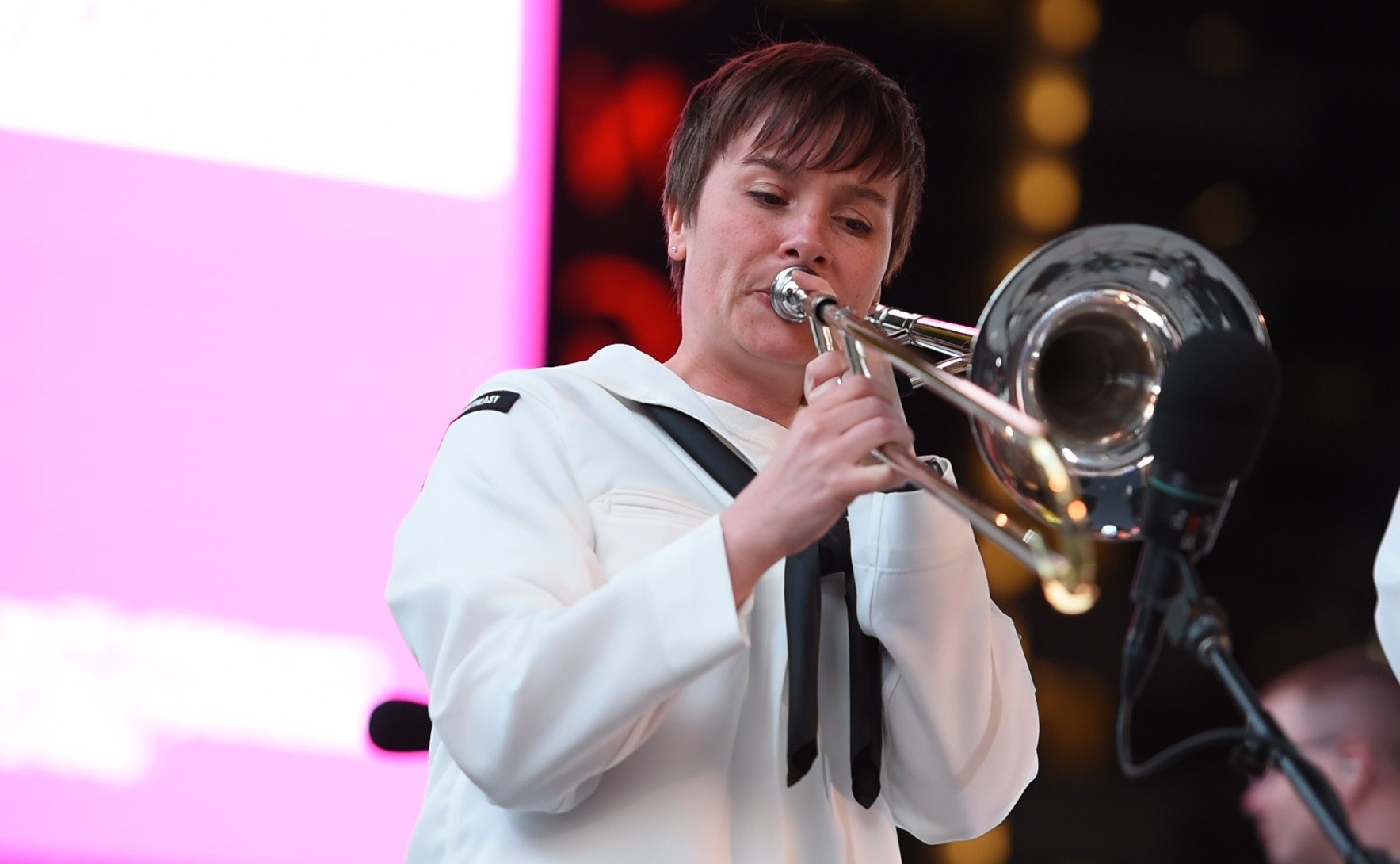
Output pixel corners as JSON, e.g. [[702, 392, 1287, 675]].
[[1121, 326, 1281, 698]]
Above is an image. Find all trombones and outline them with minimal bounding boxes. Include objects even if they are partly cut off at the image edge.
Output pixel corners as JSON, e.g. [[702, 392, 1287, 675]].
[[771, 217, 1282, 619]]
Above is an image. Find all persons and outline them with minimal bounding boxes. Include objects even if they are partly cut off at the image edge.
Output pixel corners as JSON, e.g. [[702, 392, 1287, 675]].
[[1243, 490, 1400, 864], [387, 43, 1039, 864]]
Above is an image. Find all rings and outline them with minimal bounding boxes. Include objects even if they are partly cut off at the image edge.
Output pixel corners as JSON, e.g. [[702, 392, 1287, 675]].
[[838, 374, 842, 384]]
